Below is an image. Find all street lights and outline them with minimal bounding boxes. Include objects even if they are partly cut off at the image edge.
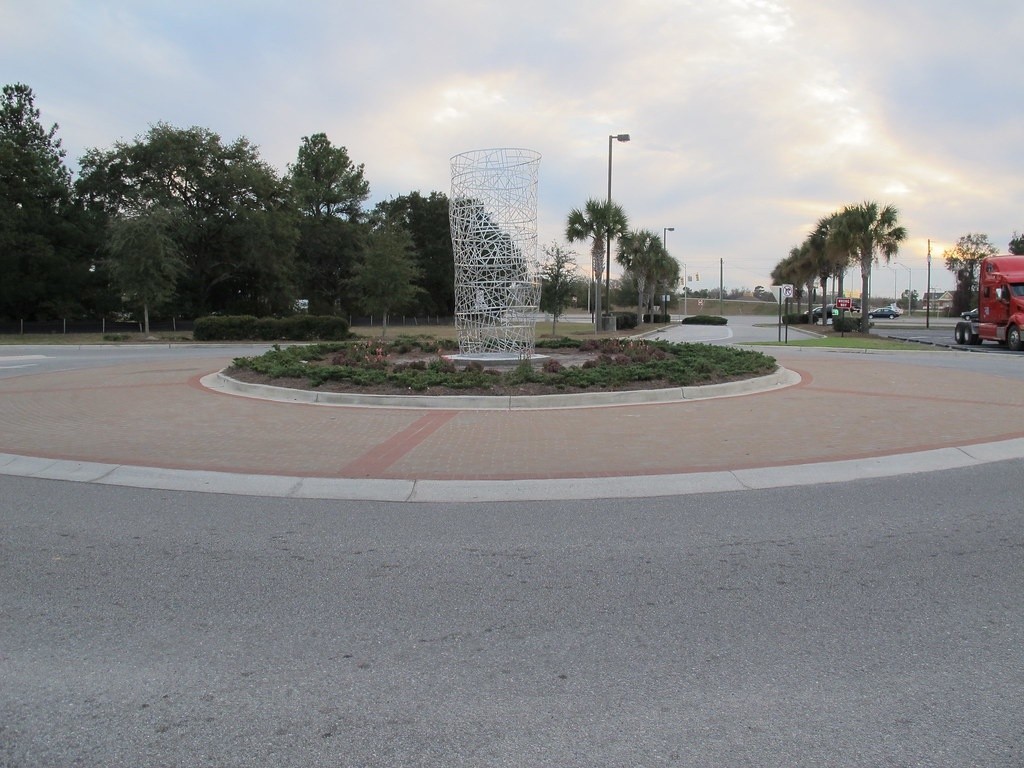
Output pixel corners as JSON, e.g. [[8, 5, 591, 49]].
[[882, 266, 896, 306], [894, 262, 911, 317], [663, 227, 674, 323], [606, 134, 631, 314]]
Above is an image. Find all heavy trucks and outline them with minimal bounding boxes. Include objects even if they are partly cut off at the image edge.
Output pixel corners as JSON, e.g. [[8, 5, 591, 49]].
[[955, 255, 1024, 351]]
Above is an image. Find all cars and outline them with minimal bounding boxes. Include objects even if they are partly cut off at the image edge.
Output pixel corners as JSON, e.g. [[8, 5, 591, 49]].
[[804, 307, 823, 318], [959, 308, 978, 321], [868, 308, 900, 320], [887, 306, 903, 315], [827, 303, 860, 313]]
